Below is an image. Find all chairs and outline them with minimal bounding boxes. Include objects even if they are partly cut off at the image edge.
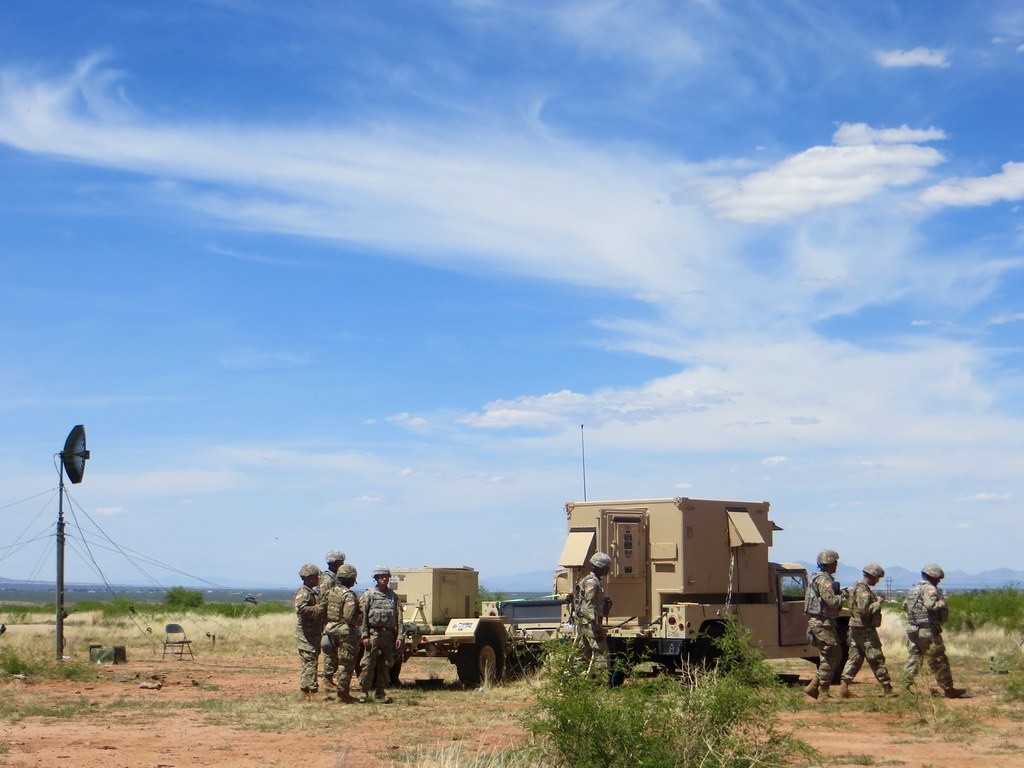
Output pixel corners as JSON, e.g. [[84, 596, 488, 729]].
[[162, 624, 195, 660]]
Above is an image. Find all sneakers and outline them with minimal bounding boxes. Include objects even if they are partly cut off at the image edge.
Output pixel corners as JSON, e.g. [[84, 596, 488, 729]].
[[945, 688, 966, 698], [335, 695, 360, 705], [300, 690, 329, 702]]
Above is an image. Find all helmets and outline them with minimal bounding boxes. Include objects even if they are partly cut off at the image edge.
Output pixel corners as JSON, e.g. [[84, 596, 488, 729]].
[[862, 562, 885, 578], [325, 550, 346, 563], [372, 565, 392, 578], [590, 552, 612, 569], [817, 550, 839, 563], [299, 564, 322, 577], [337, 565, 357, 578], [921, 563, 944, 579]]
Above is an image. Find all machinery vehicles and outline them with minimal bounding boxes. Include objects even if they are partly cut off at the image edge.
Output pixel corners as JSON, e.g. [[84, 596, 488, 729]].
[[553, 497, 821, 680]]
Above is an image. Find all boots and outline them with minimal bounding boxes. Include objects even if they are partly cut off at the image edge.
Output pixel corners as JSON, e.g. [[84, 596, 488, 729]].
[[884, 683, 900, 698], [359, 687, 369, 703], [374, 687, 393, 704], [839, 680, 858, 698], [321, 675, 337, 692], [804, 676, 835, 700]]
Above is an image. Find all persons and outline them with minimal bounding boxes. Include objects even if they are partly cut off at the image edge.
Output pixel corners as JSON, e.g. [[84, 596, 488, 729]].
[[840, 563, 899, 699], [804, 549, 849, 701], [295, 563, 323, 693], [902, 563, 966, 698], [575, 552, 612, 686], [318, 550, 363, 704], [359, 564, 403, 704]]
